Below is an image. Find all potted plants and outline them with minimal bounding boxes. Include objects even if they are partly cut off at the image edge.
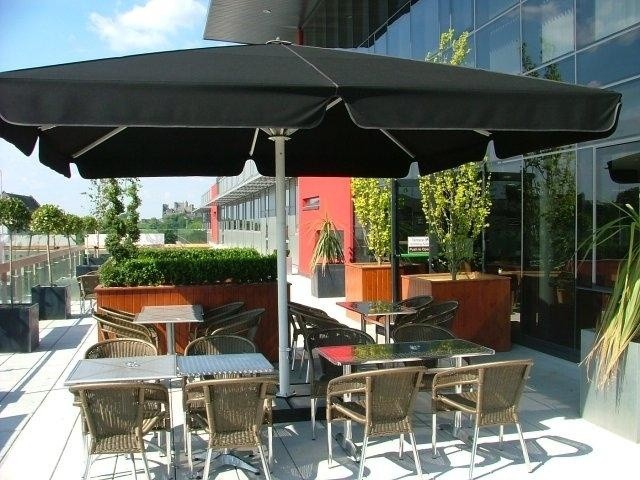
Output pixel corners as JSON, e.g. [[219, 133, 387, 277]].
[[344, 154, 513, 354], [0, 195, 71, 353], [305, 212, 346, 298]]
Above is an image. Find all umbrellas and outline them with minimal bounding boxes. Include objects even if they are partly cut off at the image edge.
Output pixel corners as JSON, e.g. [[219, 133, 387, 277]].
[[0, 37, 623, 396]]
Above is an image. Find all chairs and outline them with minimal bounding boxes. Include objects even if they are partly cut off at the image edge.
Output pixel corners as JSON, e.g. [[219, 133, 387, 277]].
[[287, 294, 460, 376], [391, 324, 472, 396], [324, 365, 427, 480], [76, 271, 99, 318], [68, 301, 280, 480], [306, 328, 375, 442], [431, 359, 535, 479]]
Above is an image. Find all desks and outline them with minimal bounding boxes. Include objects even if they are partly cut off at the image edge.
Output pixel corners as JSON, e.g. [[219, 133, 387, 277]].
[[314, 337, 497, 460]]
